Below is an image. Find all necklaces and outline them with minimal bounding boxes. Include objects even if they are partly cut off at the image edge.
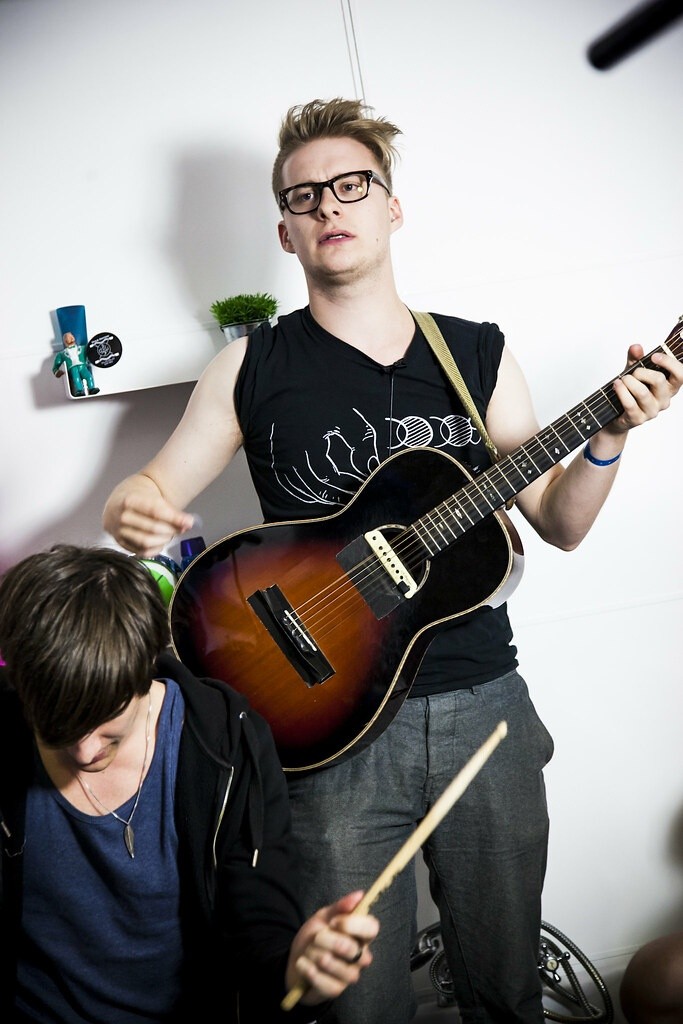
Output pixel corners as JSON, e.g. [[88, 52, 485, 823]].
[[66, 691, 152, 859]]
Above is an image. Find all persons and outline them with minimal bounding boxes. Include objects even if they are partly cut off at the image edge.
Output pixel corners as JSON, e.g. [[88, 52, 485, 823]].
[[102, 98, 683, 1024], [0, 543, 382, 1024], [619, 932, 683, 1024], [52, 332, 100, 397]]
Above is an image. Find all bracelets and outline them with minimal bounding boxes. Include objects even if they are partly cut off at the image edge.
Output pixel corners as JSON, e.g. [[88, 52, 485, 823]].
[[583, 440, 623, 466]]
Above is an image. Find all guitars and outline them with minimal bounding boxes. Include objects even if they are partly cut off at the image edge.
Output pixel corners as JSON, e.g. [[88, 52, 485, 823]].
[[165, 306, 683, 778]]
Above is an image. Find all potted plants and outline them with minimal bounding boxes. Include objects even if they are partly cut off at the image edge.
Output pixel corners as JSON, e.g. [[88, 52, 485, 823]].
[[209, 292, 279, 344]]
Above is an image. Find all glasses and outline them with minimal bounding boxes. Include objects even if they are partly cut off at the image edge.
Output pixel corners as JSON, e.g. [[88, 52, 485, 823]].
[[277, 167, 392, 215]]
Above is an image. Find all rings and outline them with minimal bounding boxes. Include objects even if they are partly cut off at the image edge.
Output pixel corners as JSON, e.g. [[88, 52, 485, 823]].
[[346, 936, 364, 964]]
